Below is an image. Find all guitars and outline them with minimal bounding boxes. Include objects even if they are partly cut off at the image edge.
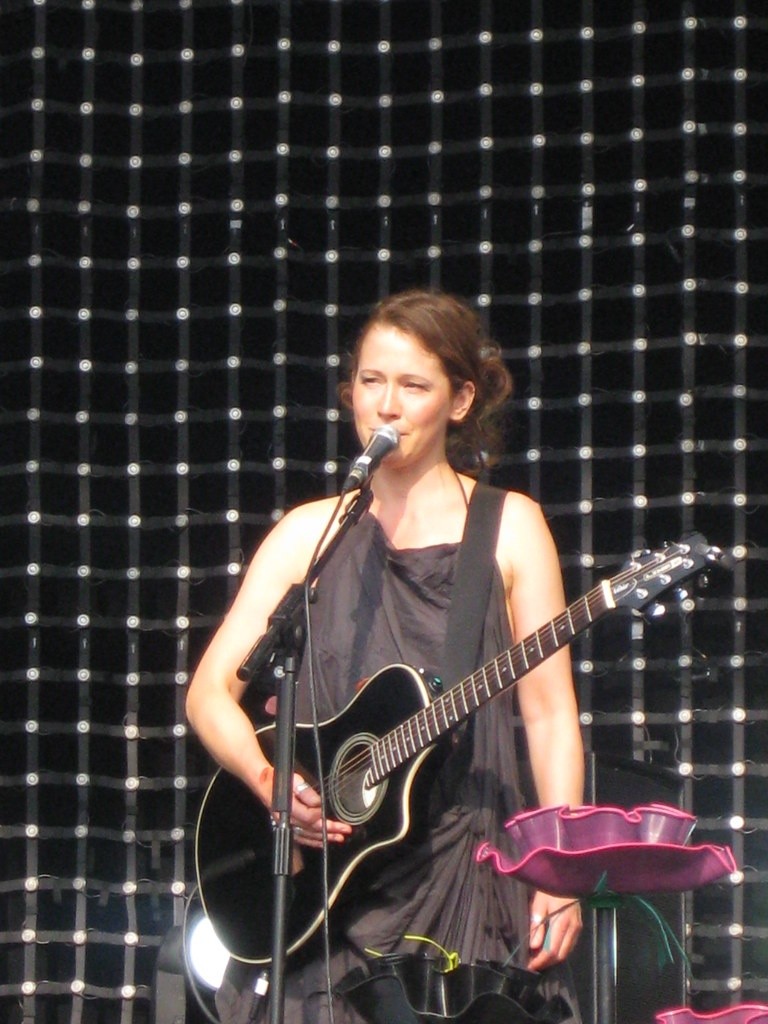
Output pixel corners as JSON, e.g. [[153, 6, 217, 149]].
[[194, 530, 715, 965]]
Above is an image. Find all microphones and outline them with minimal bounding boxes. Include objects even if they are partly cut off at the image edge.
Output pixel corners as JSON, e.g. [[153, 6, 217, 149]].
[[342, 425, 400, 491]]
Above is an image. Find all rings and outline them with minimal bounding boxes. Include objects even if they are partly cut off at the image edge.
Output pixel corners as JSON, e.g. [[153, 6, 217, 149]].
[[292, 782, 311, 796], [530, 915, 547, 924], [292, 825, 305, 835]]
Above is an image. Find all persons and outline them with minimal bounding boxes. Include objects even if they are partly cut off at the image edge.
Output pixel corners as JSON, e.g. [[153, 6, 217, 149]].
[[184, 287, 586, 1020]]
